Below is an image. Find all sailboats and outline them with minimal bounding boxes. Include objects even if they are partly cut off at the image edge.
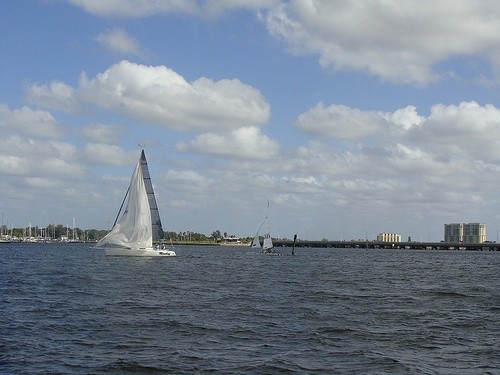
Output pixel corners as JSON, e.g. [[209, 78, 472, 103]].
[[93, 148, 177, 256], [249, 201, 283, 256]]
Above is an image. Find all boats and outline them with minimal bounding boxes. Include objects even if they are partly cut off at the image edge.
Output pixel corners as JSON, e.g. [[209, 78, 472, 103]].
[[220, 241, 251, 247]]
